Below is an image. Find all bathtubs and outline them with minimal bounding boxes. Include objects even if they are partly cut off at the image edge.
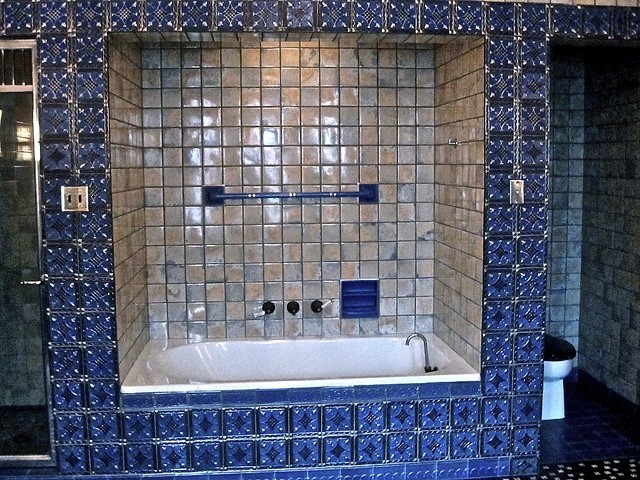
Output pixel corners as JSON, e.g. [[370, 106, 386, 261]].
[[121, 332, 480, 393]]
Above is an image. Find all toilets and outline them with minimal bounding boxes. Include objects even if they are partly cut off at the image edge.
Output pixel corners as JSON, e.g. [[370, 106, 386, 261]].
[[542, 333, 576, 420]]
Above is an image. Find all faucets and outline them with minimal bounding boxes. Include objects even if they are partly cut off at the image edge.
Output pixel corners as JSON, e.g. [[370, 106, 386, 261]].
[[405, 331, 437, 372]]
